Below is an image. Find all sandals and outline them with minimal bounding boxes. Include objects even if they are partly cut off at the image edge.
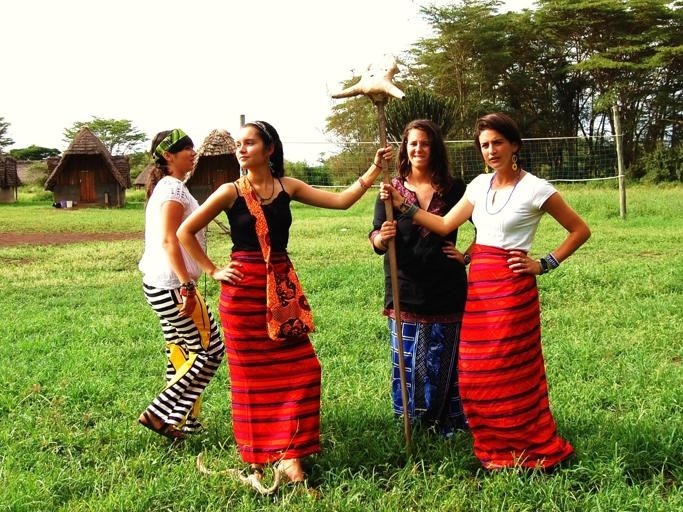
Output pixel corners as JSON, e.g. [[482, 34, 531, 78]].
[[138, 411, 185, 439]]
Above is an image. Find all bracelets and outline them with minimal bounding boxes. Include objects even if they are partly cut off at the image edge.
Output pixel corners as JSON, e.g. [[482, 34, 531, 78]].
[[537, 252, 560, 275], [210, 266, 217, 274], [179, 289, 196, 296], [373, 162, 382, 169], [404, 204, 417, 218], [359, 177, 368, 190], [378, 110, 592, 470], [180, 280, 195, 290], [400, 197, 413, 213]]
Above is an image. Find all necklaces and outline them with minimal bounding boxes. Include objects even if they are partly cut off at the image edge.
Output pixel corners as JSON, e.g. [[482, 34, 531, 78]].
[[248, 177, 275, 204], [485, 167, 521, 214]]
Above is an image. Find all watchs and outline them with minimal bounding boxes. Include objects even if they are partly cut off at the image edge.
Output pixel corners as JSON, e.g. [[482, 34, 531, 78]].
[[464, 252, 471, 264]]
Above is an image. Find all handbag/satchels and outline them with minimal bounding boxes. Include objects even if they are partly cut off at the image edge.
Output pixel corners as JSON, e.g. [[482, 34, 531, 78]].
[[266, 264, 315, 342]]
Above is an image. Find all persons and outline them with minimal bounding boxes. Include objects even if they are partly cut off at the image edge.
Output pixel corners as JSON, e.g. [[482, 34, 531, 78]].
[[176, 120, 393, 477], [367, 119, 477, 432], [135, 129, 226, 439]]
